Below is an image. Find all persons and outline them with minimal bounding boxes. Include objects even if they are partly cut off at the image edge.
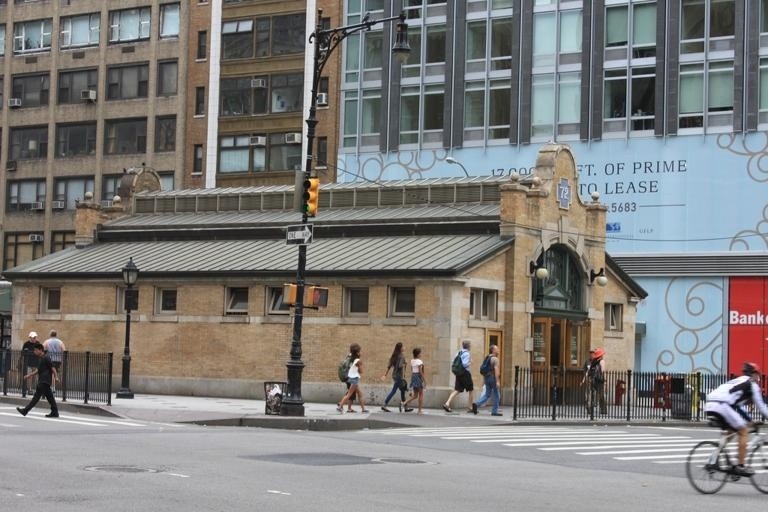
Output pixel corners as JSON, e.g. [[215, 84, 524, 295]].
[[472, 345, 504, 416], [42, 330, 65, 394], [20, 331, 44, 395], [337, 343, 369, 414], [581, 348, 607, 414], [443, 339, 479, 413], [16, 343, 59, 417], [700, 362, 768, 474], [399, 348, 426, 415], [380, 342, 414, 412]]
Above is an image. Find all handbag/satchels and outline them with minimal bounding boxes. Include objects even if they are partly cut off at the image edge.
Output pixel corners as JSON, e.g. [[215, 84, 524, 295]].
[[398, 379, 408, 391]]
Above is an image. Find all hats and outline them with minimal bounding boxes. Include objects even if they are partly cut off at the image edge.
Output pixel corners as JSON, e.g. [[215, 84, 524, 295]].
[[28, 331, 38, 338], [593, 348, 606, 360]]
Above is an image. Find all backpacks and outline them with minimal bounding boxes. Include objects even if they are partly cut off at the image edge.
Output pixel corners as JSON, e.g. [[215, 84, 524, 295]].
[[338, 356, 352, 383], [452, 351, 467, 376], [480, 354, 496, 376]]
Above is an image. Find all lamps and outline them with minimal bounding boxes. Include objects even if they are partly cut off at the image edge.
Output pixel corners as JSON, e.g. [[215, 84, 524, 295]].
[[530, 259, 548, 279], [446, 157, 469, 178], [590, 267, 607, 287]]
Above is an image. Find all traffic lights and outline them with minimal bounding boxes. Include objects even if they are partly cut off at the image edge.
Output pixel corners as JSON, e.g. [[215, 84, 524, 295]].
[[302, 175, 320, 218]]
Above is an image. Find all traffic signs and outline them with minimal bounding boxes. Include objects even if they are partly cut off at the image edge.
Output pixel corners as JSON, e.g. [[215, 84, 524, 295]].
[[285, 223, 313, 247]]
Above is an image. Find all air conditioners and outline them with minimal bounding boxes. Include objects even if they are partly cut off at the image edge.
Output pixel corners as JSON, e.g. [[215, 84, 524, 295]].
[[250, 79, 265, 88], [51, 201, 64, 209], [316, 93, 328, 105], [7, 98, 22, 107], [249, 136, 266, 147], [285, 133, 302, 144], [32, 202, 44, 210], [81, 90, 96, 100]]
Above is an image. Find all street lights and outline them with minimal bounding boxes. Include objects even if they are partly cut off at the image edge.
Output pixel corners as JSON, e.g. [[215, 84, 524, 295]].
[[111, 254, 140, 400], [275, 5, 415, 419]]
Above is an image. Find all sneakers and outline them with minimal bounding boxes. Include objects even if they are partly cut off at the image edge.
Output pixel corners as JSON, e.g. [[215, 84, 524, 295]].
[[381, 401, 426, 414], [46, 413, 59, 417], [16, 407, 27, 416], [443, 403, 502, 416], [336, 404, 371, 414], [735, 465, 755, 475]]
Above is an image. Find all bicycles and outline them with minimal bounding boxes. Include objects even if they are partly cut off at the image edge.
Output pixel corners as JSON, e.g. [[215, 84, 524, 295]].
[[682, 414, 767, 497]]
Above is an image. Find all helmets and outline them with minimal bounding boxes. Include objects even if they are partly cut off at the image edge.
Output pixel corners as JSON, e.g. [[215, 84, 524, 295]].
[[745, 360, 761, 379]]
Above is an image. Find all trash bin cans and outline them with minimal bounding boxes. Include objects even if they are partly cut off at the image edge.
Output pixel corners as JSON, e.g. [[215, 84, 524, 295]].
[[264, 381, 287, 415], [667, 384, 694, 420]]
